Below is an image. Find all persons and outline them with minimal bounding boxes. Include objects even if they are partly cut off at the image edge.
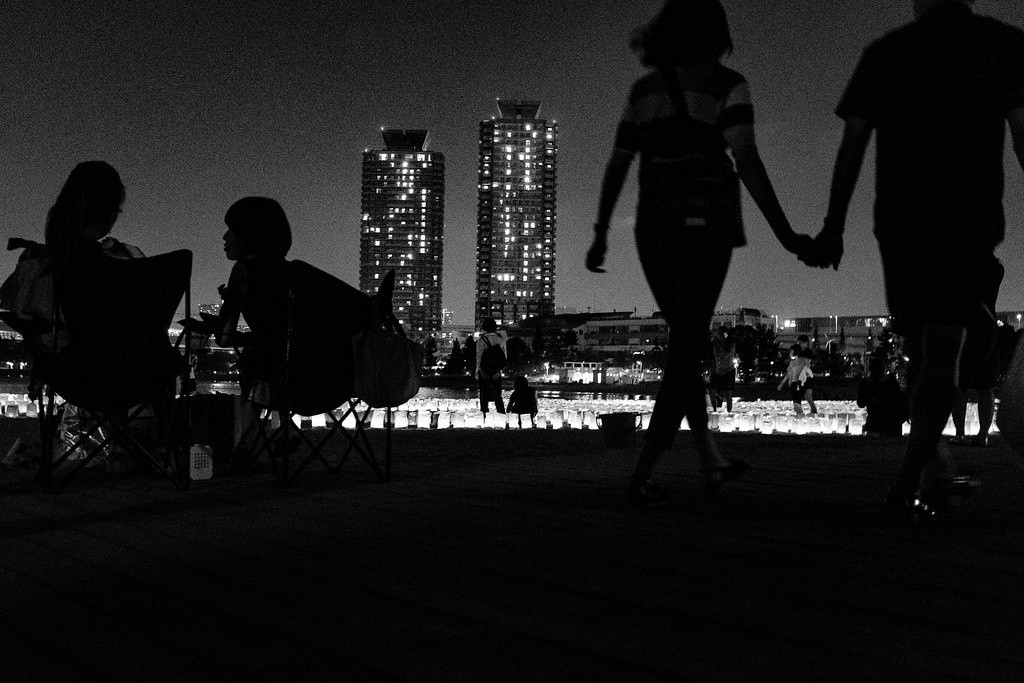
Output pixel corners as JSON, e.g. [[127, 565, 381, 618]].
[[777, 335, 817, 415], [856, 355, 905, 436], [475, 317, 538, 428], [26, 160, 185, 474], [215, 196, 356, 464], [710, 326, 736, 418], [948, 248, 1005, 447], [807, 0, 1024, 519], [584, 0, 818, 507]]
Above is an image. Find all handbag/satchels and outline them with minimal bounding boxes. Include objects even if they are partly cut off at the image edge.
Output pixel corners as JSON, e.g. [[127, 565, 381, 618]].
[[481, 335, 505, 376], [351, 296, 424, 407]]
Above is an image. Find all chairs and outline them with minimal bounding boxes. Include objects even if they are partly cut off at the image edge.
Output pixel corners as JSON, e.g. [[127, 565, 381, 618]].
[[0, 244, 193, 497], [230, 266, 396, 494]]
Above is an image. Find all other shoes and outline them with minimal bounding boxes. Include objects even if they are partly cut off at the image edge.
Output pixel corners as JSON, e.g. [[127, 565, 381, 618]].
[[948, 436, 966, 445], [929, 476, 975, 495], [885, 504, 937, 524], [704, 458, 748, 489], [629, 484, 670, 509], [969, 436, 988, 447]]
